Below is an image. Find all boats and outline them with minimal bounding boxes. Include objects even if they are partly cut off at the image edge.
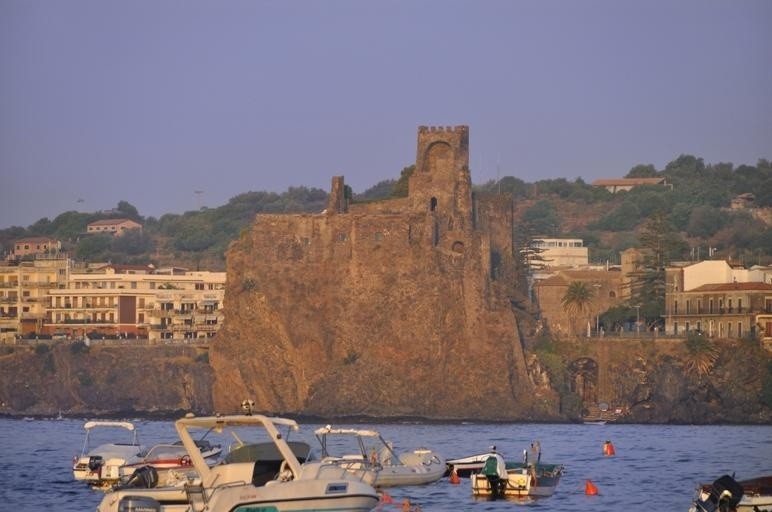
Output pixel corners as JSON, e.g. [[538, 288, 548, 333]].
[[74, 421, 221, 492], [690, 477, 772, 512], [315, 427, 446, 487], [98, 416, 377, 512], [472, 464, 563, 497], [446, 456, 494, 479]]
